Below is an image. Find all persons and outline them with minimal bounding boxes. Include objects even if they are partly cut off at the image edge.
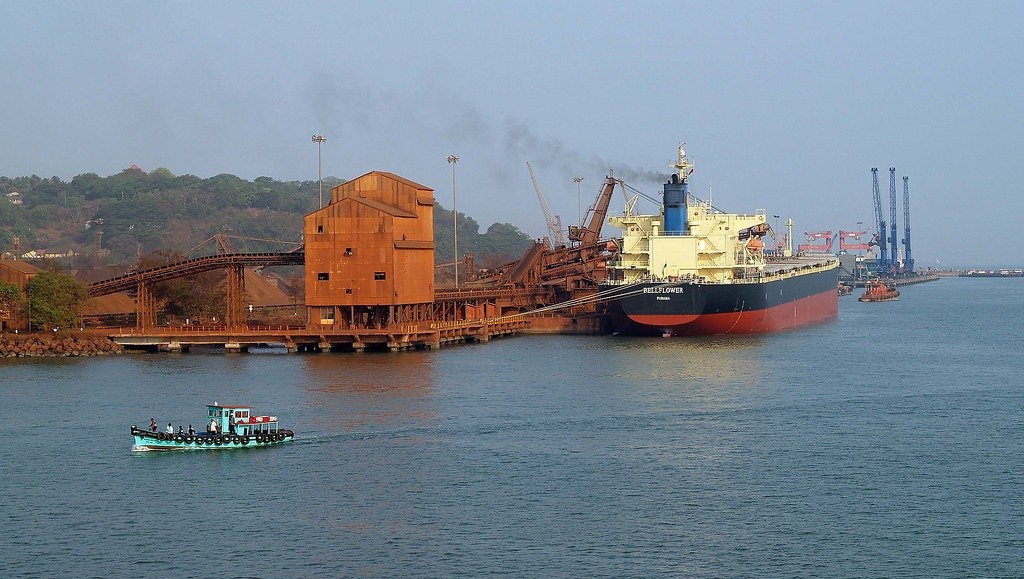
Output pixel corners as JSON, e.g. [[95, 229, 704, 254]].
[[229, 413, 235, 434], [211, 419, 218, 434], [166, 422, 174, 440], [355, 312, 362, 323], [150, 417, 157, 432], [179, 424, 195, 435], [662, 264, 668, 280]]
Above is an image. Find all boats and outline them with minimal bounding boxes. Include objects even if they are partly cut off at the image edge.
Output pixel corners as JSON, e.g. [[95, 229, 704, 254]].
[[131, 404, 295, 452], [858, 279, 900, 303], [604, 144, 839, 338]]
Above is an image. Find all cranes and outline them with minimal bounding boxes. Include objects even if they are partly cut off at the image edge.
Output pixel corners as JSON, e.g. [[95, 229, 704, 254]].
[[526, 160, 569, 248], [868, 166, 914, 278]]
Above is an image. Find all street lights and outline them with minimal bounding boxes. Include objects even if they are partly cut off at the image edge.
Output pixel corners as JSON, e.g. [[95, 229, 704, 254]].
[[773, 215, 780, 235], [573, 177, 584, 227], [857, 221, 864, 278], [312, 135, 328, 210], [447, 154, 460, 288]]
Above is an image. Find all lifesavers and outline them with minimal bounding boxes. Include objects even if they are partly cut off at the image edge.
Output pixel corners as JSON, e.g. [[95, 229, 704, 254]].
[[264, 434, 272, 443], [175, 434, 185, 443], [214, 436, 223, 445], [278, 432, 285, 441], [233, 435, 241, 444], [157, 431, 166, 440], [240, 435, 250, 444], [223, 434, 232, 444], [256, 433, 264, 443], [185, 435, 194, 444], [195, 436, 204, 445], [167, 433, 174, 441], [272, 433, 279, 442], [206, 435, 214, 445]]
